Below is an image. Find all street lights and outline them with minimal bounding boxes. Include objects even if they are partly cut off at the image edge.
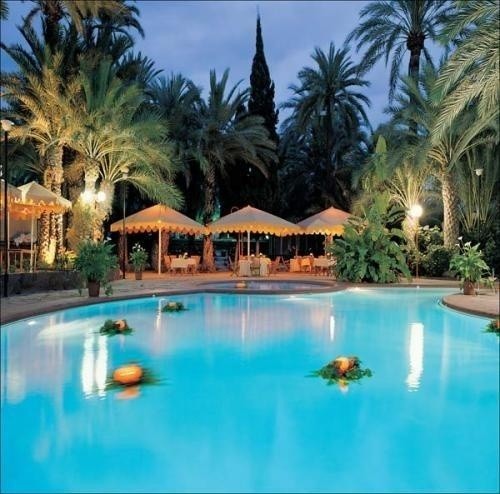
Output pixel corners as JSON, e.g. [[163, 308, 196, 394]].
[[411, 204, 423, 279], [474, 168, 483, 247], [120, 166, 130, 279], [1, 120, 14, 298]]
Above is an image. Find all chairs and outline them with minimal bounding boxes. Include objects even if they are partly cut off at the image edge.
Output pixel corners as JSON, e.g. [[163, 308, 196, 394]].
[[164, 254, 203, 276], [282, 255, 338, 275], [227, 256, 280, 280]]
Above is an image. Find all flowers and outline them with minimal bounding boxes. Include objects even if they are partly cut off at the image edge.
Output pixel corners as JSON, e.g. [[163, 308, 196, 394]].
[[452, 235, 490, 284]]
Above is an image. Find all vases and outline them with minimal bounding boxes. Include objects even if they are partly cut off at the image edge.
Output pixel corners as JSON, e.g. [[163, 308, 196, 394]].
[[129, 241, 150, 271], [463, 280, 477, 296], [135, 270, 142, 280]]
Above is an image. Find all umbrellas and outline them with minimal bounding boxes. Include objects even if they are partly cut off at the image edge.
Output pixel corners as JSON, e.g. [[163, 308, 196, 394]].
[[296, 206, 389, 257], [10, 181, 73, 272], [0, 179, 22, 273], [110, 202, 208, 275], [207, 204, 303, 256]]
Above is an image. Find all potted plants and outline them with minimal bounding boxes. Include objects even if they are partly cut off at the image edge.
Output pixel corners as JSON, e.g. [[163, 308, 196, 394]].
[[73, 235, 118, 295]]
